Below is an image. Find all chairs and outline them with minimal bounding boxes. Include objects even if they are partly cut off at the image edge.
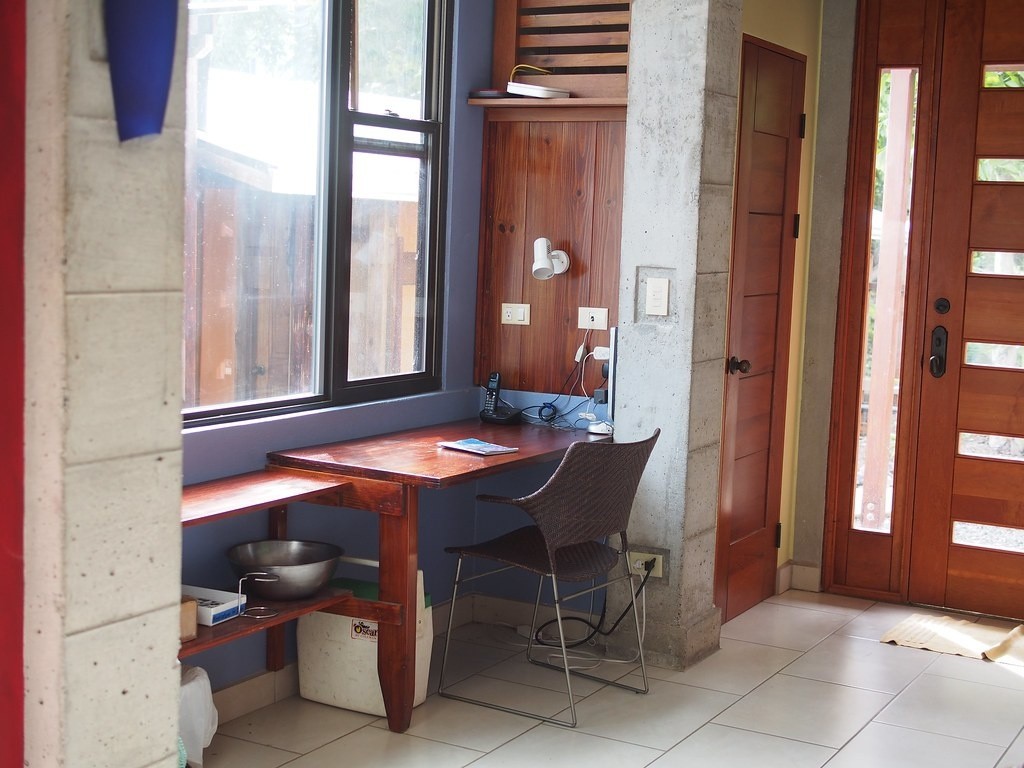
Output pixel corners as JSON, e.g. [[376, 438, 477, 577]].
[[437, 427, 661, 727]]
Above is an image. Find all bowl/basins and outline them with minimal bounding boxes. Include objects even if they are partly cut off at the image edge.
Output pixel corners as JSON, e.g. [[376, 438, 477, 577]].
[[227, 539, 345, 600]]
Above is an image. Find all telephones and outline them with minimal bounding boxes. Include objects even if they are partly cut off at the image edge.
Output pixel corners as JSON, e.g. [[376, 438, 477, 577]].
[[480, 372, 523, 426]]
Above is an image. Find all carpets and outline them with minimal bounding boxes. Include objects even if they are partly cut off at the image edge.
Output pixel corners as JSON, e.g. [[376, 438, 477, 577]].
[[876, 611, 1023, 665]]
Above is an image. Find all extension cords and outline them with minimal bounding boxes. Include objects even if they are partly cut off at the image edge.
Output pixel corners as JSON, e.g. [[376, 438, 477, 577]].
[[608, 326, 617, 421]]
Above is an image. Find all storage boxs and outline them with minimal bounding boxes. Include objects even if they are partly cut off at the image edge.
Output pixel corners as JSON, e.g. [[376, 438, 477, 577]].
[[178, 594, 200, 645], [180, 584, 247, 627], [294, 554, 434, 717]]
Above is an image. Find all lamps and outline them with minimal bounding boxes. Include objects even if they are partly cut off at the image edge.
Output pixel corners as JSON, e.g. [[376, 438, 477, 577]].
[[531, 237, 570, 279]]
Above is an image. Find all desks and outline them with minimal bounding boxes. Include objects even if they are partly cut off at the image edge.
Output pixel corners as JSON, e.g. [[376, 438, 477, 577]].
[[262, 416, 618, 735]]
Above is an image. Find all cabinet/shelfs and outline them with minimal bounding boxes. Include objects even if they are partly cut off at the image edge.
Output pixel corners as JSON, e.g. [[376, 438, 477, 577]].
[[180, 468, 352, 661]]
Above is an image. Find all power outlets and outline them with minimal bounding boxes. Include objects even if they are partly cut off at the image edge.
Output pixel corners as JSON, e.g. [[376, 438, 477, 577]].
[[501, 302, 531, 325], [627, 551, 664, 578]]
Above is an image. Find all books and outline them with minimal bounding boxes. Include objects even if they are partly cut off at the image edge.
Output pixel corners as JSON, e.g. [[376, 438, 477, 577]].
[[438, 438, 519, 455]]
